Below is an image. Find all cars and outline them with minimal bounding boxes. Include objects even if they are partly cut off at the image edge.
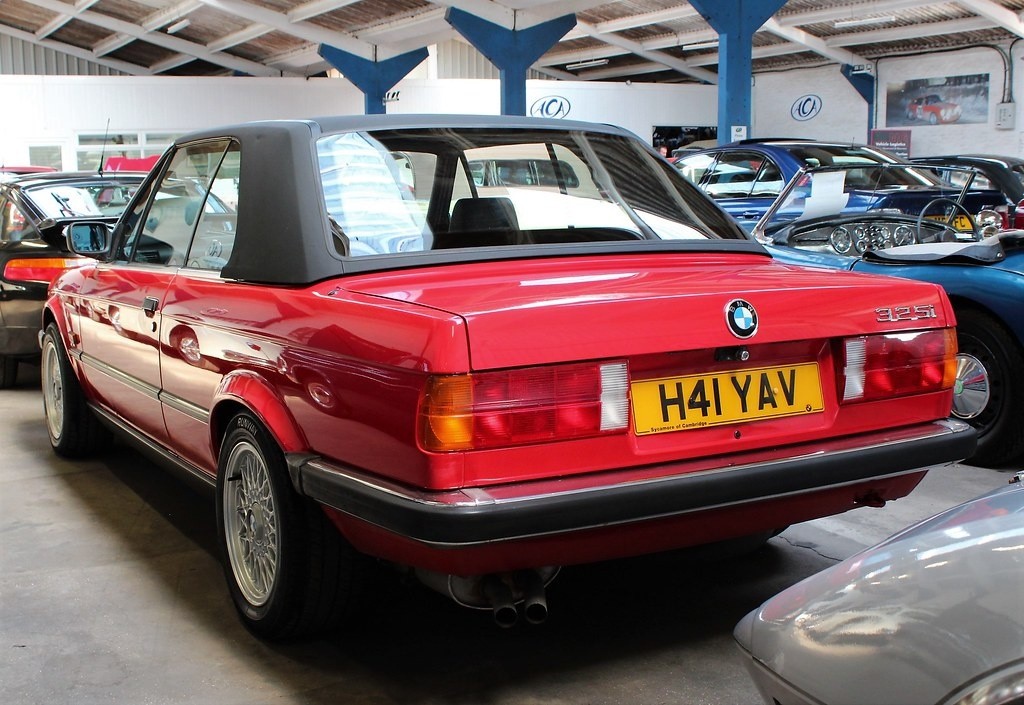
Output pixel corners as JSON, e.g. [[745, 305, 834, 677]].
[[1, 168, 238, 384], [670, 135, 1006, 233], [38, 114, 980, 639], [750, 161, 1023, 467], [385, 142, 708, 241]]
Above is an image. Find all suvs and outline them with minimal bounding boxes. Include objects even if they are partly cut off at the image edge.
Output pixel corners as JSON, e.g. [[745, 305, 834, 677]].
[[907, 151, 1022, 207]]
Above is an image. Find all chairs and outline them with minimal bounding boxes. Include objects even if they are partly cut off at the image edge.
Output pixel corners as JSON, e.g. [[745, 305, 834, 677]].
[[724, 164, 782, 197], [449, 198, 519, 232]]
